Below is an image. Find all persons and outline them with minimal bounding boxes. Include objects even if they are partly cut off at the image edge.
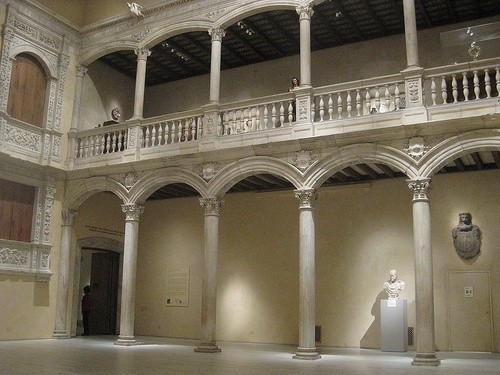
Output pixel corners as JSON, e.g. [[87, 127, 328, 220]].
[[288, 78, 302, 120], [81, 285, 92, 336], [452, 210, 482, 259], [384, 270, 405, 298]]
